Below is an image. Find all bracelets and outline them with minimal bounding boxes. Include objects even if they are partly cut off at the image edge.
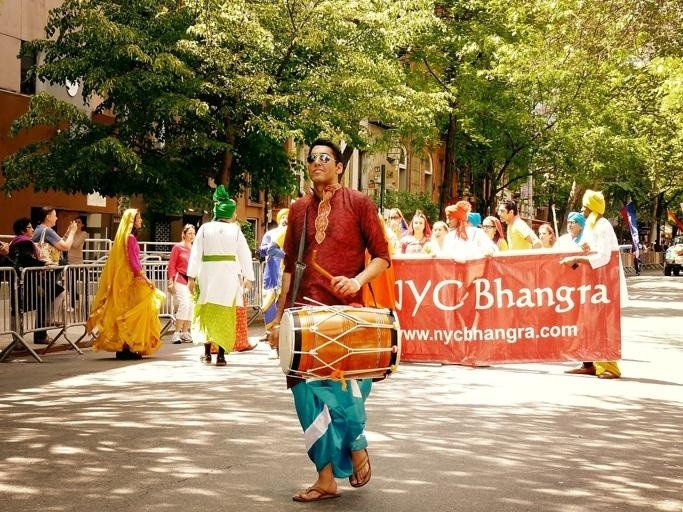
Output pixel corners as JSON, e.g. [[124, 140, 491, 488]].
[[349, 277, 360, 292], [62, 229, 68, 238], [167, 283, 173, 289]]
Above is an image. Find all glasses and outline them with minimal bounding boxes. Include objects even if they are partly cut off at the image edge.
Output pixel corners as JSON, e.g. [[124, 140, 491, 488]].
[[307, 154, 333, 163]]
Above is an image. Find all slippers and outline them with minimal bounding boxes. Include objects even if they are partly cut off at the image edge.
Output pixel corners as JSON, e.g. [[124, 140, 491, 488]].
[[349, 449, 371, 488], [294, 487, 341, 502]]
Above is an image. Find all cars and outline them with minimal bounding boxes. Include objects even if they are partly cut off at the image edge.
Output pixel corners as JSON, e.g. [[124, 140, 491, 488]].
[[662, 236, 683, 276], [620, 243, 643, 253]]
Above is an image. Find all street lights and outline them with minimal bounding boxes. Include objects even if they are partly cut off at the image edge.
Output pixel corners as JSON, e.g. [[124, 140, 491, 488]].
[[526, 102, 557, 231], [379, 158, 399, 211]]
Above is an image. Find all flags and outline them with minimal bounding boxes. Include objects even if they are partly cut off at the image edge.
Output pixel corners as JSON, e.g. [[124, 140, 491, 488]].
[[665, 209, 682, 233], [619, 203, 642, 272]]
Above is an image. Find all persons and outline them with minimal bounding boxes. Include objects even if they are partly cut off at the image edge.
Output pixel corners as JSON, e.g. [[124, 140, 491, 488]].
[[618, 238, 671, 256], [8, 217, 66, 352], [261, 189, 628, 380], [167, 223, 197, 343], [186, 200, 255, 367], [267, 137, 392, 502], [0, 241, 10, 256], [29, 207, 77, 344], [211, 287, 258, 355], [86, 208, 165, 359], [66, 215, 86, 314]]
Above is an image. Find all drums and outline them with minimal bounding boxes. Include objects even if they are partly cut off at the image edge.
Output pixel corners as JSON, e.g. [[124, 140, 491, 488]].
[[278, 304, 402, 381]]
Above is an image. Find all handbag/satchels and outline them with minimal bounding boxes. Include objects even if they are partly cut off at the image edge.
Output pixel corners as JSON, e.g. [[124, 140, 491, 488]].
[[179, 271, 196, 282], [35, 243, 61, 266]]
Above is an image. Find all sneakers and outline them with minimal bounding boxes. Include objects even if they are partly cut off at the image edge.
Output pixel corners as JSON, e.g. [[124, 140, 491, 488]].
[[216, 359, 226, 365], [599, 371, 617, 379], [116, 351, 141, 360], [566, 367, 596, 375], [171, 333, 193, 343], [200, 355, 211, 363]]
